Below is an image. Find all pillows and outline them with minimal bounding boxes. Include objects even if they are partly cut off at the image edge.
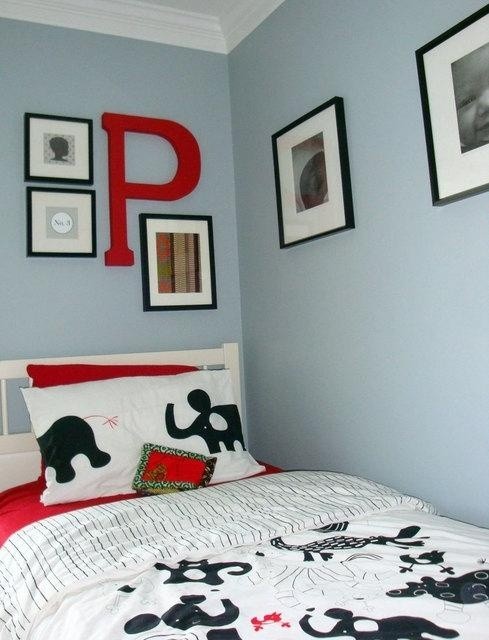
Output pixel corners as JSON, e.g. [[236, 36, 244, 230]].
[[24, 371, 264, 506], [28, 361, 206, 493]]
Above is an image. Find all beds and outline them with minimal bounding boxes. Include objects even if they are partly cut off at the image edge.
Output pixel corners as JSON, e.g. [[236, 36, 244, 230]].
[[0, 341, 489, 639]]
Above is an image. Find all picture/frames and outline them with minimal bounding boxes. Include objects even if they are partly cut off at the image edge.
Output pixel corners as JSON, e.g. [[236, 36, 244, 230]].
[[139, 213, 218, 311], [271, 95, 354, 250], [23, 112, 92, 187], [414, 3, 489, 206], [26, 187, 97, 259]]
